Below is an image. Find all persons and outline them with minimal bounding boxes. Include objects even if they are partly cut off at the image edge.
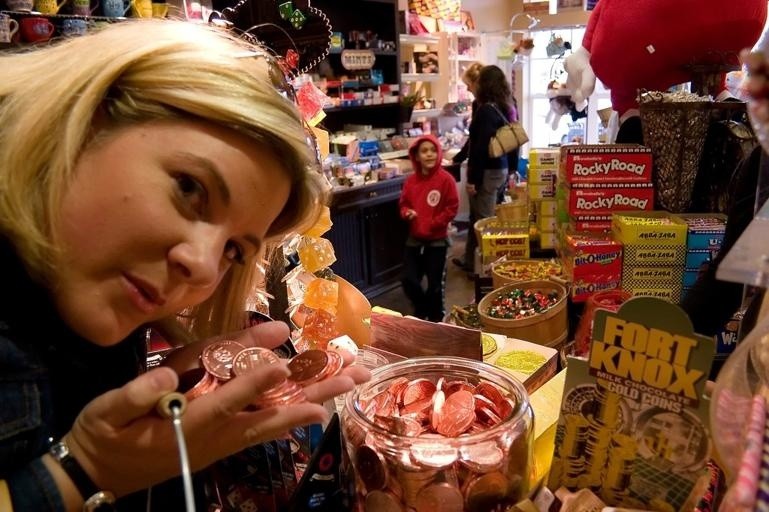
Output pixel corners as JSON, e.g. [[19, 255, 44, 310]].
[[0, 17, 371, 512], [452, 63, 518, 204], [449, 64, 518, 282], [398, 135, 460, 322]]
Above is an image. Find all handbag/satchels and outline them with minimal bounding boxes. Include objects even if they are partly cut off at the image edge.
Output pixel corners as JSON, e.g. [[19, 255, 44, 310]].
[[484, 102, 531, 158]]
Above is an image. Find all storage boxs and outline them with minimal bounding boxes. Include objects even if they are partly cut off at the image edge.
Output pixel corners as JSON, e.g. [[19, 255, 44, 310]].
[[472, 140, 731, 348]]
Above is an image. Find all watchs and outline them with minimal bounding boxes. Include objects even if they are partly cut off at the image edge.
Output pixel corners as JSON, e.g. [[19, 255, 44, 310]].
[[50, 440, 116, 512]]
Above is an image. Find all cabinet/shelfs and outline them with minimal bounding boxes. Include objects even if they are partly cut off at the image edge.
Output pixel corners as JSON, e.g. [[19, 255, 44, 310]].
[[298, 0, 523, 299]]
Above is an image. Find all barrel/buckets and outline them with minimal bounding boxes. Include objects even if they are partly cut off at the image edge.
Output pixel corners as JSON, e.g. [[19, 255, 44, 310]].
[[479, 277, 571, 350]]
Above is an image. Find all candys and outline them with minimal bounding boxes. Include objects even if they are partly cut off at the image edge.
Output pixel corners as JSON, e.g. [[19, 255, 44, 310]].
[[175, 259, 564, 510]]
